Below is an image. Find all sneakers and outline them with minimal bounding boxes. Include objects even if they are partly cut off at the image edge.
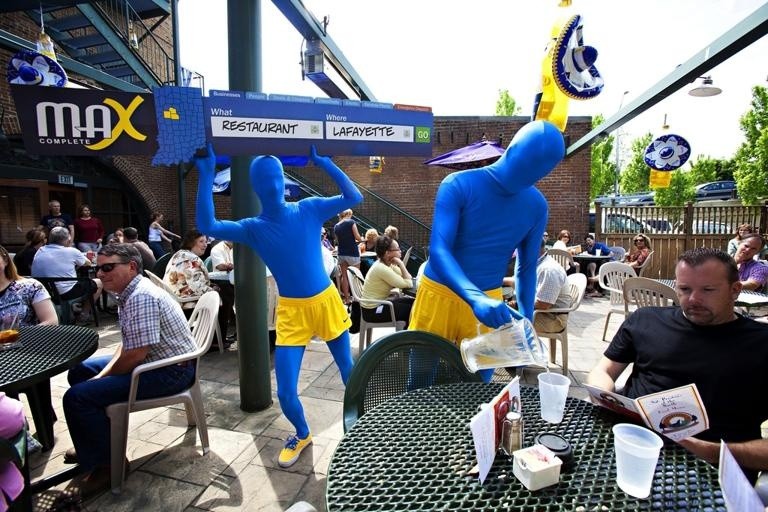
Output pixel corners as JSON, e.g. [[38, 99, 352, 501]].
[[278, 432, 312, 468], [71, 302, 95, 326]]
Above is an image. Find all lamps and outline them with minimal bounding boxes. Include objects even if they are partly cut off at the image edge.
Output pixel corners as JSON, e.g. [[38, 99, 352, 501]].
[[676, 64, 722, 97]]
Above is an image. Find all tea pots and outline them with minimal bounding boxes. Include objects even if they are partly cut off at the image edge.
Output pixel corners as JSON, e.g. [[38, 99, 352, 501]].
[[459, 312, 551, 374]]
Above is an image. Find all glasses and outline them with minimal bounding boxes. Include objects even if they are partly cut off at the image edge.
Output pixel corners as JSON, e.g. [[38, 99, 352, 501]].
[[95, 261, 139, 272]]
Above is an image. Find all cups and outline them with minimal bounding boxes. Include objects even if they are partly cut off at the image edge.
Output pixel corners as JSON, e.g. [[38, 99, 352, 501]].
[[0, 309, 21, 349], [570, 245, 604, 257], [536, 371, 574, 426], [612, 421, 664, 500]]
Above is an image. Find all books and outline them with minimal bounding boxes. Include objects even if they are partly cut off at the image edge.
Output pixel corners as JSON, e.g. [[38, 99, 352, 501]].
[[582, 382, 710, 444]]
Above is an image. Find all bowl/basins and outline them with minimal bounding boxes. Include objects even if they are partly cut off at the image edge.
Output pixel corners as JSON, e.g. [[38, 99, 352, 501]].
[[533, 432, 575, 472]]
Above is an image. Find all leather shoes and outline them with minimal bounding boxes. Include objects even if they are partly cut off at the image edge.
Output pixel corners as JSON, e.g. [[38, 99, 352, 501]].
[[60, 456, 130, 502], [64, 447, 77, 460]]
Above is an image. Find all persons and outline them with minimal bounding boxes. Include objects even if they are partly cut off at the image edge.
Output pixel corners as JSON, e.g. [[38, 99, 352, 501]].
[[406, 119, 565, 391], [194, 141, 364, 467], [2, 200, 279, 512], [734, 232, 768, 293], [584, 248, 768, 487], [502, 229, 652, 335], [321, 208, 428, 331], [726, 224, 768, 257]]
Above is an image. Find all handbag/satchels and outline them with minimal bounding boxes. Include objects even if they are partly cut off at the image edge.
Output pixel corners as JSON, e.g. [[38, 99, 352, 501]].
[[349, 301, 360, 334]]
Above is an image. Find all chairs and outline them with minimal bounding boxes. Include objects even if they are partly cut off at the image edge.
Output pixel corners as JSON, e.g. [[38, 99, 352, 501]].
[[621, 275, 678, 322], [1, 407, 32, 511], [6, 277, 61, 456], [531, 271, 589, 378], [98, 289, 221, 496], [20, 243, 223, 354], [598, 260, 638, 341], [347, 246, 657, 277], [346, 265, 406, 357]]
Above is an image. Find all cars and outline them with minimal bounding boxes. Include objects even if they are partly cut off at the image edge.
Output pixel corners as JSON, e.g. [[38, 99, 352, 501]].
[[692, 180, 738, 201], [593, 191, 657, 206], [670, 220, 736, 235]]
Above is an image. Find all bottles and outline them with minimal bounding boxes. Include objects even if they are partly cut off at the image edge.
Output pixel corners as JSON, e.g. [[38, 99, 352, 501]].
[[502, 410, 524, 457]]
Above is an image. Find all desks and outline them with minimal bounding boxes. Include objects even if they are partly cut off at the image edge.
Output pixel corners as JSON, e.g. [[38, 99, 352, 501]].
[[324, 381, 730, 511], [640, 276, 768, 315]]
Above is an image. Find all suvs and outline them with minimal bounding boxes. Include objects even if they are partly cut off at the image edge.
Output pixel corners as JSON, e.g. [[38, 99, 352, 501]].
[[587, 208, 657, 234], [635, 216, 672, 235]]
